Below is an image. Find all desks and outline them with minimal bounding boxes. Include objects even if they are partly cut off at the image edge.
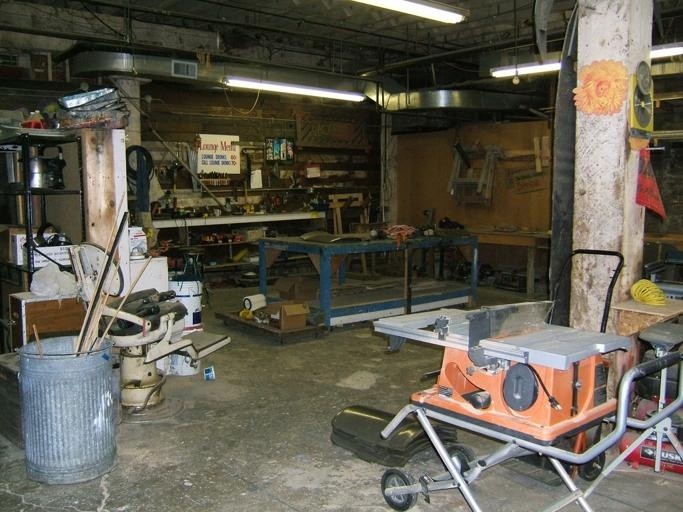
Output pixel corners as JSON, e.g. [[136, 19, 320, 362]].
[[472, 225, 550, 298], [258, 233, 477, 333]]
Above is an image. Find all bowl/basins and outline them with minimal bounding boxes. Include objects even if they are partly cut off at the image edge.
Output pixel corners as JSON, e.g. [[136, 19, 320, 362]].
[[24, 236, 73, 247]]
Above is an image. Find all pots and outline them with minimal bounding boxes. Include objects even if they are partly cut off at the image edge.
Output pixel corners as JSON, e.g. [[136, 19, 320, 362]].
[[0, 145, 65, 190]]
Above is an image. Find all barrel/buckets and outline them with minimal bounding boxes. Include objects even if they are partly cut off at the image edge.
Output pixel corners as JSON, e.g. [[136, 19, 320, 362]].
[[170, 280, 203, 330]]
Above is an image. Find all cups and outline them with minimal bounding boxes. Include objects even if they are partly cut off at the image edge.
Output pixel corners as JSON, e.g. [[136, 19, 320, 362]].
[[203, 365, 215, 381], [131, 247, 144, 257], [131, 238, 138, 245]]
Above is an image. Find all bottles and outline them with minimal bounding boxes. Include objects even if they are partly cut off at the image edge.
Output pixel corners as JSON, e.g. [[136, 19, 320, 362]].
[[275, 192, 281, 208], [223, 198, 232, 216], [28, 110, 46, 129]]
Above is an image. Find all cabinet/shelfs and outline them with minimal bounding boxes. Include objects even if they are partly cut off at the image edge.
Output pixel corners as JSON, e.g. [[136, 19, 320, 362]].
[[153, 212, 329, 267]]
